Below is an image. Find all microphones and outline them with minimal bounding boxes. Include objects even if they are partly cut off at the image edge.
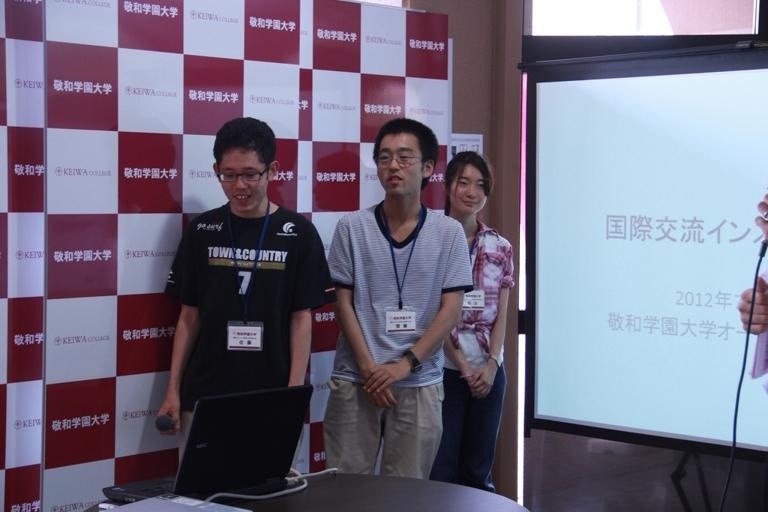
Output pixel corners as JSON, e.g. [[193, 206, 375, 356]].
[[155, 412, 177, 438]]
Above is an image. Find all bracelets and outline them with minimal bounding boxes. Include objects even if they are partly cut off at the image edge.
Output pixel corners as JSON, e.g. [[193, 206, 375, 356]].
[[403, 348, 422, 374], [488, 353, 502, 368], [459, 372, 475, 379]]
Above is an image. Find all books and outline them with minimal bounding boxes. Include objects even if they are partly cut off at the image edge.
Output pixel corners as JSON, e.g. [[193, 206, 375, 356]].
[[750, 332, 768, 378]]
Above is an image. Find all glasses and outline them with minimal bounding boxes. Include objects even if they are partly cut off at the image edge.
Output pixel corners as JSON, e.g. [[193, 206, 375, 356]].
[[218, 165, 269, 181], [376, 155, 423, 167]]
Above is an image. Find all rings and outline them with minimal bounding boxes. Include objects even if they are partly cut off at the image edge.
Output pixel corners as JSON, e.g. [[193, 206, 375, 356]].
[[762, 210, 768, 219]]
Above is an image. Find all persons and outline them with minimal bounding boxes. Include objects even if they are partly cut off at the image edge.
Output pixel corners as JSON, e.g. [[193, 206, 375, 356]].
[[734, 194, 768, 336], [326, 118, 476, 483], [154, 118, 339, 475], [443, 149, 514, 498]]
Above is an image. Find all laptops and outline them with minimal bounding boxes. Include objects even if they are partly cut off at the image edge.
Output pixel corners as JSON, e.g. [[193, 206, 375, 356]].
[[101, 382, 315, 502]]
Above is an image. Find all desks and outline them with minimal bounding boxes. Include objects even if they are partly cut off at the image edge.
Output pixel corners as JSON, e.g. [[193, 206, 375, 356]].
[[83, 471, 531, 512]]
